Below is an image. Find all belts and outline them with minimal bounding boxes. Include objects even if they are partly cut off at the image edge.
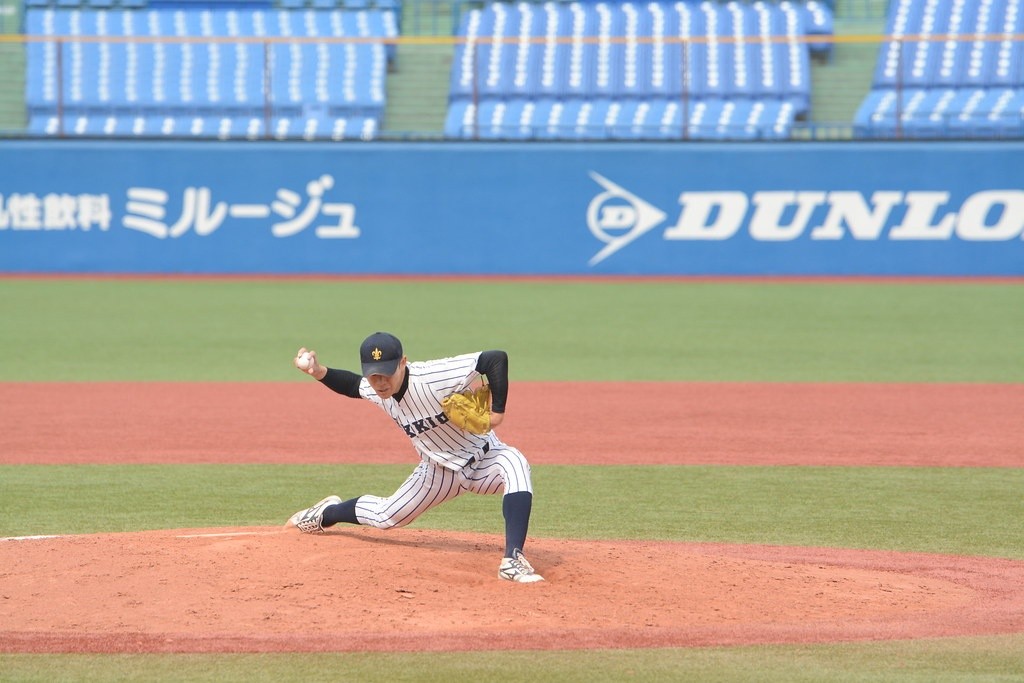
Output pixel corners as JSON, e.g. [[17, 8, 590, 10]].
[[465, 442, 489, 467]]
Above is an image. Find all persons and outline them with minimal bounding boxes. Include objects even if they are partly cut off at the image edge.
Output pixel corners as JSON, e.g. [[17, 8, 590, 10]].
[[283, 332, 543, 582]]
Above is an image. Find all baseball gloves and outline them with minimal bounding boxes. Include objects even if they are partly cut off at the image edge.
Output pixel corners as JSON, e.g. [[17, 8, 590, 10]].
[[438, 382, 494, 436]]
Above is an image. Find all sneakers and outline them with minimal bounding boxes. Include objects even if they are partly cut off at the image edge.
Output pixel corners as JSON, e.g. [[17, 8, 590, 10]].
[[283, 495, 342, 535], [498, 547, 544, 582]]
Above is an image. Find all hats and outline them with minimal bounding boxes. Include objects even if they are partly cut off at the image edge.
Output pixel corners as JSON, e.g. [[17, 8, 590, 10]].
[[360, 332, 403, 378]]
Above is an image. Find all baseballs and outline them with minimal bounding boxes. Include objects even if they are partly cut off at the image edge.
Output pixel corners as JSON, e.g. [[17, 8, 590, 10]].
[[296, 351, 315, 371]]
